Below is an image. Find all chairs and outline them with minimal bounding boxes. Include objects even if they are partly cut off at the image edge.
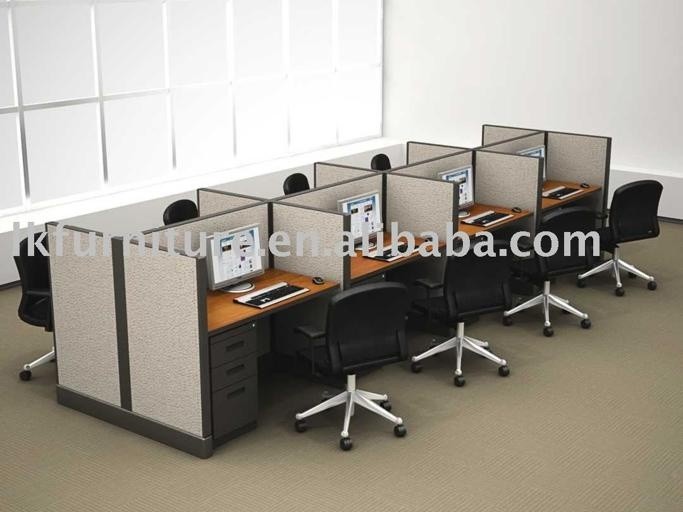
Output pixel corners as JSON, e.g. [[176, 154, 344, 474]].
[[500, 207, 593, 336], [294, 283, 411, 450], [413, 239, 510, 385], [14, 232, 56, 381], [578, 179, 663, 296]]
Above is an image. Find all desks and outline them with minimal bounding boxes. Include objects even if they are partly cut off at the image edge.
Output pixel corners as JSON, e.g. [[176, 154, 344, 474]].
[[119, 200, 351, 458], [406, 123, 545, 179], [197, 162, 386, 237], [473, 133, 613, 274], [314, 141, 472, 181], [386, 150, 541, 329], [270, 172, 458, 375], [46, 188, 268, 422]]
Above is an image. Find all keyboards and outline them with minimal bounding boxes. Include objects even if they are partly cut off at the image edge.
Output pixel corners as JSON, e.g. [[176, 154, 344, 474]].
[[363, 240, 422, 263], [461, 210, 514, 227], [233, 281, 311, 309], [542, 185, 584, 200]]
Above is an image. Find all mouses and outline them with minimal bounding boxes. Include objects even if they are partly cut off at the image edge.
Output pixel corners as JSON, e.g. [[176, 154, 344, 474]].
[[581, 183, 589, 188], [512, 207, 521, 213], [312, 276, 324, 285], [421, 238, 432, 243]]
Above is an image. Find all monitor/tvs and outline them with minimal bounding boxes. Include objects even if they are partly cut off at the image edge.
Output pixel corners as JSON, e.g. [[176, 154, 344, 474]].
[[437, 165, 474, 218], [516, 145, 547, 191], [204, 223, 264, 293], [336, 190, 384, 250]]
